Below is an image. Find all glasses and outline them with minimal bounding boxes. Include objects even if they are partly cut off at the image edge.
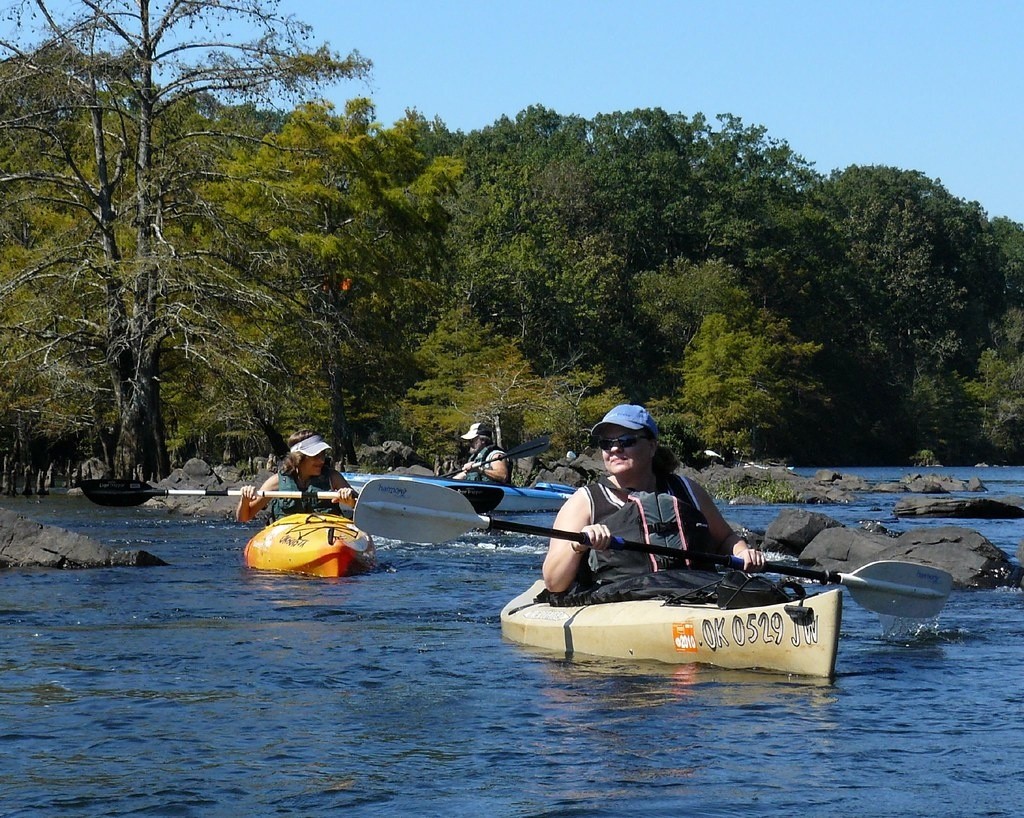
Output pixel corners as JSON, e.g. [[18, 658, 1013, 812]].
[[597, 433, 650, 450]]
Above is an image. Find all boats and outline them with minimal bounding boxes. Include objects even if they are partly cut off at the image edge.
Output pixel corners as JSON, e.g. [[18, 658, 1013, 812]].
[[338, 471, 578, 515], [243, 511, 377, 578], [500, 587, 843, 679]]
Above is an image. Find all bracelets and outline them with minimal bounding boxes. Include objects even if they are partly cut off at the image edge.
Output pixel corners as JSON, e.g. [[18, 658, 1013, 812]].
[[477, 467, 484, 474], [571, 543, 584, 554]]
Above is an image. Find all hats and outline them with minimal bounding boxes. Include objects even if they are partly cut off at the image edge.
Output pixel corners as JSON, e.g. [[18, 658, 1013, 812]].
[[591, 403, 659, 439], [289, 434, 332, 457], [460, 423, 492, 439]]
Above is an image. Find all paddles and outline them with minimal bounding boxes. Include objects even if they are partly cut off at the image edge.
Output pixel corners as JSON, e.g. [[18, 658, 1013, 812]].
[[353, 480, 955, 619], [79, 478, 505, 515], [441, 436, 549, 478]]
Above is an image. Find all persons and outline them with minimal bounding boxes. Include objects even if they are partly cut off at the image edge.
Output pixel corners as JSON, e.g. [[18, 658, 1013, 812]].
[[236, 431, 359, 526], [541, 404, 766, 604], [452, 423, 513, 485]]
